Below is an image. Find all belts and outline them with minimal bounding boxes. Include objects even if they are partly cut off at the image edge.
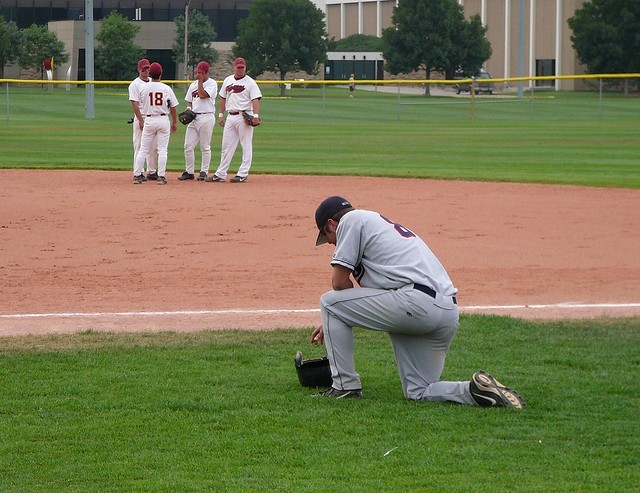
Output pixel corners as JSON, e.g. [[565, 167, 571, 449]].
[[147, 114, 166, 117], [229, 110, 245, 115], [414, 283, 456, 303], [194, 113, 207, 114]]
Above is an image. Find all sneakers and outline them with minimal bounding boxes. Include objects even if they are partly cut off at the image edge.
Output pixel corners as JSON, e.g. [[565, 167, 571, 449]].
[[178, 171, 194, 180], [147, 174, 157, 180], [230, 176, 247, 183], [313, 387, 363, 399], [140, 174, 147, 181], [469, 371, 526, 408], [197, 172, 207, 180], [156, 176, 166, 185], [133, 176, 142, 183], [205, 175, 226, 182]]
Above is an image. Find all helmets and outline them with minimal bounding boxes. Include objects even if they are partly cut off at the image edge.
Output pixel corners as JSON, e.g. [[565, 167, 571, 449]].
[[294, 351, 333, 388]]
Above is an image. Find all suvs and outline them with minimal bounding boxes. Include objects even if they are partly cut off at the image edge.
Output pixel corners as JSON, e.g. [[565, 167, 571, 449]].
[[455, 72, 493, 95]]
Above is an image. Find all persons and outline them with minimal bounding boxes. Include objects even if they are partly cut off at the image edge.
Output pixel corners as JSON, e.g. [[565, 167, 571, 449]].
[[204, 57, 262, 183], [128, 58, 159, 181], [132, 63, 179, 184], [303, 196, 527, 411], [177, 60, 219, 179], [348, 73, 356, 97]]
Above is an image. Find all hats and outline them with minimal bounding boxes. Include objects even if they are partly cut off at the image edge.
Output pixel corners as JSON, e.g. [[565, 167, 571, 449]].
[[314, 195, 351, 246], [137, 59, 150, 69], [194, 61, 209, 73], [234, 57, 246, 67], [148, 63, 163, 75]]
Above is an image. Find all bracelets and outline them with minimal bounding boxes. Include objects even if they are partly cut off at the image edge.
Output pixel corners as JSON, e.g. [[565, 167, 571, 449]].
[[253, 113, 258, 118], [219, 112, 223, 117]]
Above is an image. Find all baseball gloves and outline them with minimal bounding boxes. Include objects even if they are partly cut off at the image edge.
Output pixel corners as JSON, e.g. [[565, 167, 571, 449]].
[[178, 109, 196, 125], [244, 114, 261, 126]]
